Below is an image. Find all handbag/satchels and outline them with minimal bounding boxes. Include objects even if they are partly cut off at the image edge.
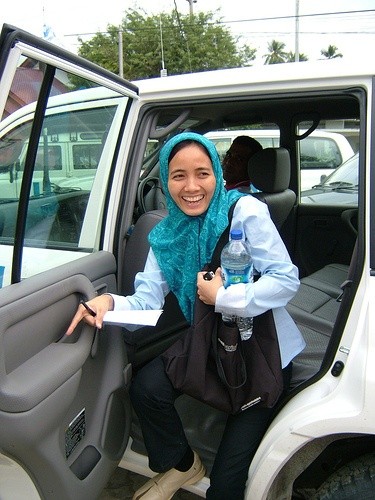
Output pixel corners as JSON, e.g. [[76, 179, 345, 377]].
[[166, 198, 284, 410]]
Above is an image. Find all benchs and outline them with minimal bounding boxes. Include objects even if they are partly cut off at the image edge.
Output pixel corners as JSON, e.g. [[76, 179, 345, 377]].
[[285, 264, 349, 386]]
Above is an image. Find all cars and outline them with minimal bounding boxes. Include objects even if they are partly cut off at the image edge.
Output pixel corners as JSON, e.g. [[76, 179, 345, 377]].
[[300, 151, 359, 207]]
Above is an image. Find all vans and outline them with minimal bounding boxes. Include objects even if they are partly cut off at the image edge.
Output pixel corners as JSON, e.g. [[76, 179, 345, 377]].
[[0, 21, 375, 500], [203, 129, 356, 191], [0, 131, 160, 199]]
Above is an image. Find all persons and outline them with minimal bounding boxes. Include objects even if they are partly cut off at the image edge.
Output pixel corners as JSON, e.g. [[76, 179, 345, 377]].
[[66, 132, 306, 500]]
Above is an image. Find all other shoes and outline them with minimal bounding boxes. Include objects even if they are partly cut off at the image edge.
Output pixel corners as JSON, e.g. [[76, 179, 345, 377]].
[[131, 451, 206, 500]]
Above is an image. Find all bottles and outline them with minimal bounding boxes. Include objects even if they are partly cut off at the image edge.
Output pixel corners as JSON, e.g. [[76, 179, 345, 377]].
[[222, 229, 254, 341]]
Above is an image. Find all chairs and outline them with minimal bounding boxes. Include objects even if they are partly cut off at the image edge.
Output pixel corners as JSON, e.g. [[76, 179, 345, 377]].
[[248, 148, 297, 228], [121, 208, 187, 367]]
